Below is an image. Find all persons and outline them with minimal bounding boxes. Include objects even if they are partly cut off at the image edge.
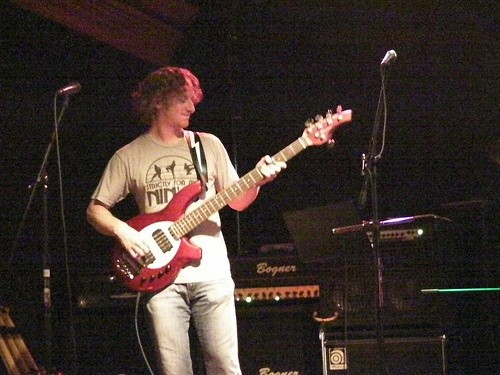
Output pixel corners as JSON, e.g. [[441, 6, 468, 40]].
[[85, 67, 287, 375]]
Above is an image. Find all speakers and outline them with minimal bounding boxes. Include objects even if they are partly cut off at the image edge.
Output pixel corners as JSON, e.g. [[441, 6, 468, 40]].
[[326, 215, 455, 333], [237, 303, 326, 375], [326, 334, 447, 375]]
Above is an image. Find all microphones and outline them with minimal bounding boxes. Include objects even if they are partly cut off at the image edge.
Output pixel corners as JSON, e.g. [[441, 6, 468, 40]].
[[58, 81, 81, 95], [381, 49, 397, 65]]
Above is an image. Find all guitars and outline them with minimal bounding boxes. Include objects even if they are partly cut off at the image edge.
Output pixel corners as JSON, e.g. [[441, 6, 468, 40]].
[[108, 103, 354, 294]]
